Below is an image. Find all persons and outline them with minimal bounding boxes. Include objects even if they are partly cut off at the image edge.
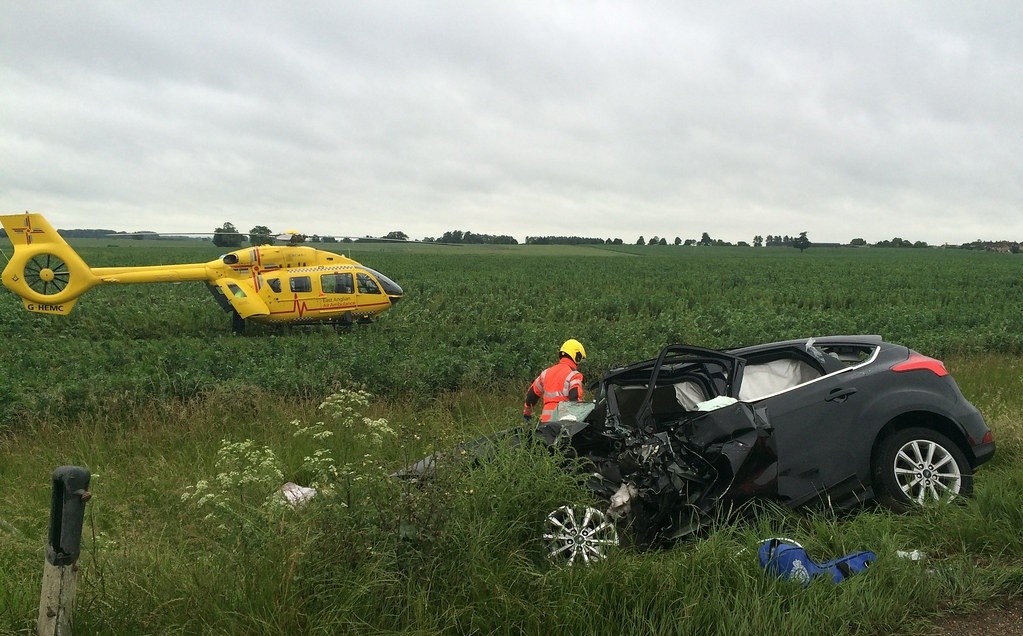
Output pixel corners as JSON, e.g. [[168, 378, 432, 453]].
[[233, 286, 245, 334], [522, 339, 586, 426]]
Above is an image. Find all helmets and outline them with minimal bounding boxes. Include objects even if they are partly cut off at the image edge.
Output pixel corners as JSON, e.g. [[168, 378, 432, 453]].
[[559, 339, 586, 365]]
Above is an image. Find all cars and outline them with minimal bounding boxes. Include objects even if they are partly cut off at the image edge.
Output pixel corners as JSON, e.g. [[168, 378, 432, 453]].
[[376, 335, 997, 581]]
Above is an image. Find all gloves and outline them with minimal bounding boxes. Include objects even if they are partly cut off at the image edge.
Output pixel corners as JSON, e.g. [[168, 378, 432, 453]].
[[523, 414, 531, 421]]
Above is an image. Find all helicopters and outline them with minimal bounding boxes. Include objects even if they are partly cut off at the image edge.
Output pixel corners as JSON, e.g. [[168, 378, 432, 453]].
[[0, 214, 406, 331]]
